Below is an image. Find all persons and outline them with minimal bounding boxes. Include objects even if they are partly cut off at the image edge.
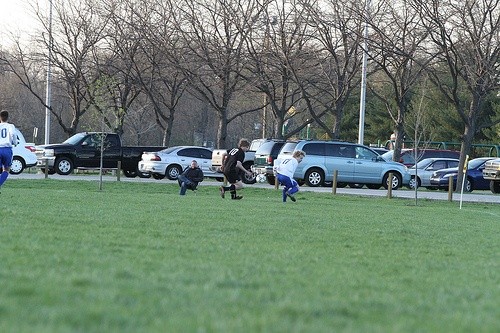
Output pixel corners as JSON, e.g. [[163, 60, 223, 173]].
[[177, 160, 204, 196], [219, 139, 253, 199], [0, 109, 17, 194], [276, 150, 306, 202]]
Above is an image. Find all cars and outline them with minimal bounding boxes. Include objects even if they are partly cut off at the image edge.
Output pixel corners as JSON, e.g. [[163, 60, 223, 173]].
[[429, 156, 497, 192], [137, 145, 225, 183], [408, 157, 460, 190]]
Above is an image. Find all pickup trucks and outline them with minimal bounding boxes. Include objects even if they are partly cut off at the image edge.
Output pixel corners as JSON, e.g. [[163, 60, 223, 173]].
[[36, 131, 169, 180], [210, 138, 268, 185], [6, 142, 38, 174]]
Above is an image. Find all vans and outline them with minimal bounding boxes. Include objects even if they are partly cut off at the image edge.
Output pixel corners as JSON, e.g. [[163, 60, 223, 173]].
[[382, 148, 459, 168]]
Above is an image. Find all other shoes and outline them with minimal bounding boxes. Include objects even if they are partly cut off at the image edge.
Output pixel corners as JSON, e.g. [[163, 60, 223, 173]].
[[191, 184, 196, 191], [235, 196, 243, 200], [219, 185, 225, 199], [285, 191, 296, 202]]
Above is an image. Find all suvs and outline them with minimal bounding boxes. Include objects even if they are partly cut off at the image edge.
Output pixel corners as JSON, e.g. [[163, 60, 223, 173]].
[[249, 138, 411, 190], [481, 157, 500, 194]]
